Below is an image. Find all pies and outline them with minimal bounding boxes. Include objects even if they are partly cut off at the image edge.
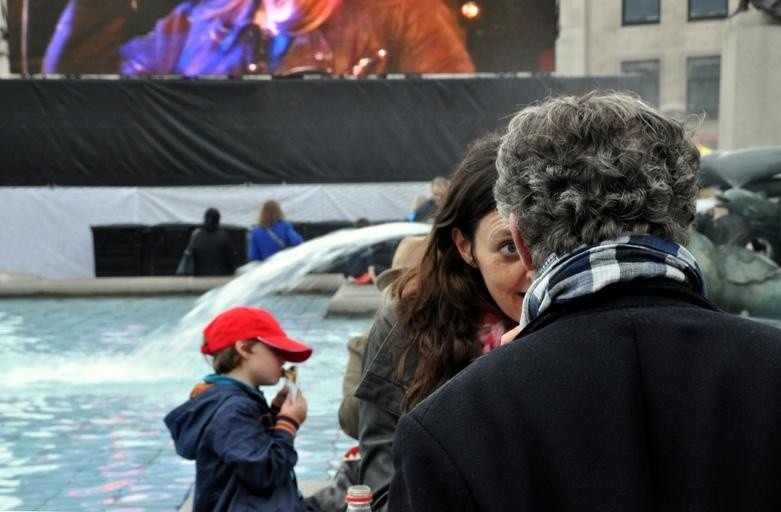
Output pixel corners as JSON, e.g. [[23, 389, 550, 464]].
[[284, 367, 297, 382]]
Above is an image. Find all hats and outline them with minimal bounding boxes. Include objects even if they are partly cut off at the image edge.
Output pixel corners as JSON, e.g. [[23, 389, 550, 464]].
[[200, 306, 312, 363]]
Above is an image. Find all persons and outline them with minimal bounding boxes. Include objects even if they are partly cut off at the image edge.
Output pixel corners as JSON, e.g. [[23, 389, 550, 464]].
[[408, 195, 428, 219], [352, 138, 537, 512], [36, 0, 478, 79], [386, 93, 781, 511], [162, 306, 317, 511], [188, 207, 238, 275], [339, 233, 434, 441], [413, 176, 448, 222], [248, 197, 306, 263]]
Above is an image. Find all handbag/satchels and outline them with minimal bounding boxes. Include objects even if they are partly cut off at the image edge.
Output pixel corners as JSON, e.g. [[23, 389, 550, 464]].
[[176, 249, 197, 276]]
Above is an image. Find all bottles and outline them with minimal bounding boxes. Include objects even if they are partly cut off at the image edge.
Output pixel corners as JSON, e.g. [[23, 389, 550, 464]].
[[346, 485, 373, 512]]
